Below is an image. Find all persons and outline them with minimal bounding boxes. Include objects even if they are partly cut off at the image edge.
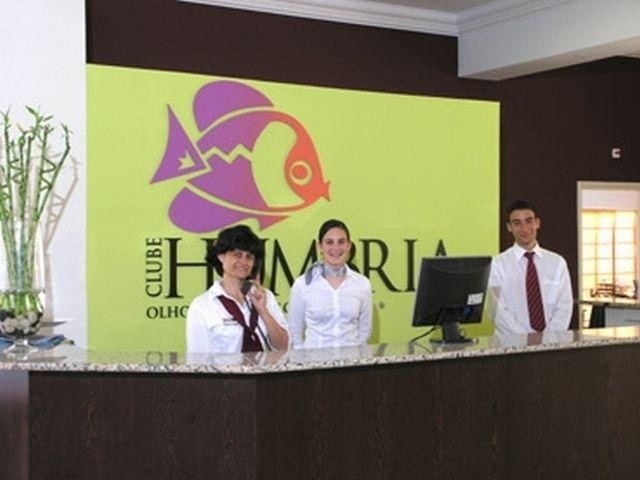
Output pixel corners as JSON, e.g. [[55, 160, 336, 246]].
[[185, 224, 296, 352], [483, 202, 574, 334], [286, 219, 373, 350]]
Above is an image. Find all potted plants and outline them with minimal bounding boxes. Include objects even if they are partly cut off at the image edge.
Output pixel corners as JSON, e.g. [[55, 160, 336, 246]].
[[0, 104, 71, 336]]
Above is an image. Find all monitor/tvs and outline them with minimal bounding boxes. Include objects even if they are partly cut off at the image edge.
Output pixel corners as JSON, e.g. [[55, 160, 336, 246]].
[[412, 256, 492, 344]]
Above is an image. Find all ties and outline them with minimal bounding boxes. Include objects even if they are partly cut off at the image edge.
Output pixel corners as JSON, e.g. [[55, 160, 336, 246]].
[[525, 253, 545, 331]]
